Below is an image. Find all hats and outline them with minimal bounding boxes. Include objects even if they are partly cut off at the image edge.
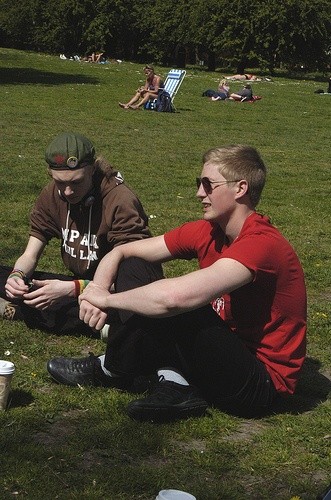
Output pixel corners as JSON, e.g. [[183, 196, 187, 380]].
[[45, 133, 96, 170]]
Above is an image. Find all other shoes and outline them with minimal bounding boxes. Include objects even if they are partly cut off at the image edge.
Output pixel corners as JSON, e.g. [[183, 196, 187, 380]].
[[241, 96, 247, 102], [0, 296, 19, 321]]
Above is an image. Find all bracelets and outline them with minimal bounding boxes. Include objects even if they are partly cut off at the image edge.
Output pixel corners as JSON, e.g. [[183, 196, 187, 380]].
[[73, 279, 90, 296], [8, 269, 25, 279]]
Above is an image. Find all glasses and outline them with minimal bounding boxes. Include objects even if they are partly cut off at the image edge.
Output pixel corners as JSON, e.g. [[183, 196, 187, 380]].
[[196, 177, 245, 194]]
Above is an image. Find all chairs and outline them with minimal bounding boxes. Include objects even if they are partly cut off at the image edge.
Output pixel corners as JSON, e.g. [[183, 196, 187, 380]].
[[151, 69, 186, 112]]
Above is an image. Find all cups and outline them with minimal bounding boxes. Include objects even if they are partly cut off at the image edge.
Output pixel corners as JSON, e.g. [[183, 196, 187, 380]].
[[0, 360, 15, 410], [155, 488, 197, 500]]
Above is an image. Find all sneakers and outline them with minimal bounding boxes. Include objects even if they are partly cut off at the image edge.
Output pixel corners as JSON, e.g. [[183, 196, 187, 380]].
[[126, 375, 209, 422], [47, 351, 136, 388]]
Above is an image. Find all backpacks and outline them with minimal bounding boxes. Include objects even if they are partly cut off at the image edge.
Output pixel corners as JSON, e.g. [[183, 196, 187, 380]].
[[156, 91, 172, 112]]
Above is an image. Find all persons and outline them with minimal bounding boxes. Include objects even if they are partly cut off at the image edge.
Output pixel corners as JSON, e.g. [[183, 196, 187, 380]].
[[47, 144, 307, 421], [118, 65, 167, 110], [210, 74, 257, 101], [88, 51, 105, 64], [0, 132, 154, 340]]
